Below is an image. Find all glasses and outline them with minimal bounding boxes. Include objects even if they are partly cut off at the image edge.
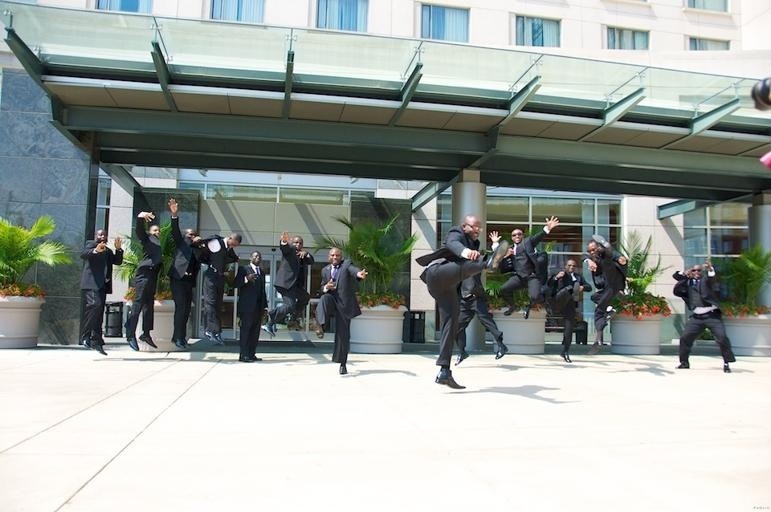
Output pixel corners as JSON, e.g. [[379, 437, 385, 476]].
[[692, 269, 701, 272], [568, 264, 576, 267]]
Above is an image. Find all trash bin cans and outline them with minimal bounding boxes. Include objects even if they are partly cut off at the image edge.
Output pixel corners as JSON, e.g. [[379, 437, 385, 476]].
[[104, 301, 123, 337], [401, 310, 425, 343], [576, 321, 588, 345]]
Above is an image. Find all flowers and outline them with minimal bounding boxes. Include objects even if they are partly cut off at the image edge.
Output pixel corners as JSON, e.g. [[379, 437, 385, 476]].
[[0, 281, 47, 300], [610, 293, 672, 320], [719, 302, 771, 319], [121, 285, 173, 301], [359, 291, 408, 309]]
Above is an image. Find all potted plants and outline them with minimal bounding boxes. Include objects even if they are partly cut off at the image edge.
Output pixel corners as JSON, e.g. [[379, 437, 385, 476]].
[[310, 209, 423, 356], [603, 227, 673, 355], [707, 242, 771, 359], [485, 226, 559, 355], [0, 212, 74, 349], [113, 218, 196, 353]]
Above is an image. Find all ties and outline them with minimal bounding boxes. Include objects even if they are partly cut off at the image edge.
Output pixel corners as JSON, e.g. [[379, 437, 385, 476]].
[[332, 266, 336, 281]]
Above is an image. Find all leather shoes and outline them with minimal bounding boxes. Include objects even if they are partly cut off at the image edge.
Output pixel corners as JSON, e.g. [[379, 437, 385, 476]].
[[90, 335, 188, 356], [312, 320, 323, 338], [573, 282, 579, 302], [724, 365, 730, 372], [261, 324, 274, 336], [562, 352, 571, 362], [592, 234, 610, 249], [206, 332, 225, 345], [679, 364, 689, 368], [487, 240, 508, 274], [496, 345, 508, 359], [436, 368, 465, 388], [504, 307, 528, 320], [340, 366, 346, 374], [240, 354, 262, 362], [455, 352, 469, 365]]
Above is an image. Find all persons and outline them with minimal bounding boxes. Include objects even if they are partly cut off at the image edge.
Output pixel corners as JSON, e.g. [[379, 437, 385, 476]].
[[455, 248, 513, 364], [193, 232, 240, 345], [233, 251, 268, 362], [124, 212, 162, 351], [489, 216, 559, 319], [548, 260, 592, 362], [583, 234, 628, 355], [80, 230, 123, 355], [673, 261, 735, 372], [167, 198, 199, 349], [315, 248, 368, 374], [415, 217, 509, 389], [268, 232, 314, 337]]
